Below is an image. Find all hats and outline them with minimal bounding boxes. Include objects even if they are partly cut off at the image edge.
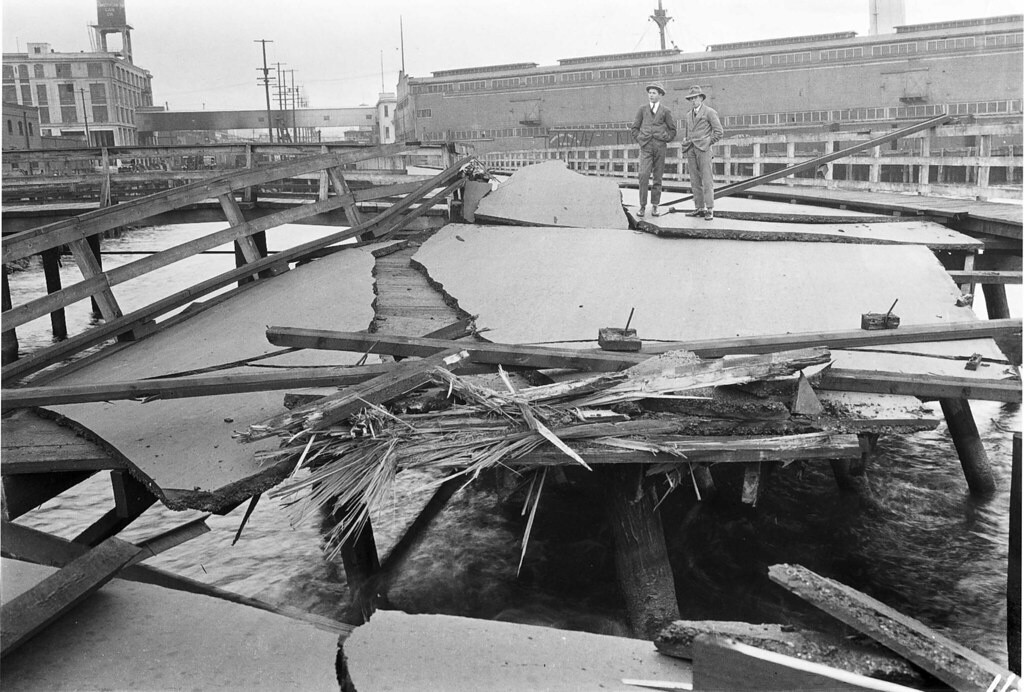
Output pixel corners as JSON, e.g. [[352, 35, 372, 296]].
[[685, 85, 706, 101], [646, 81, 665, 96]]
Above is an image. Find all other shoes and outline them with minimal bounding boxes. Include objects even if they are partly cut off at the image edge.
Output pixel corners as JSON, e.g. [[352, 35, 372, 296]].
[[685, 208, 705, 218], [705, 209, 714, 221], [651, 205, 659, 216], [636, 206, 645, 216]]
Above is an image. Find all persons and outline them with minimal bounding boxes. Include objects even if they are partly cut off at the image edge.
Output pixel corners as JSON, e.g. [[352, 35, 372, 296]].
[[631, 81, 677, 218], [682, 84, 724, 221]]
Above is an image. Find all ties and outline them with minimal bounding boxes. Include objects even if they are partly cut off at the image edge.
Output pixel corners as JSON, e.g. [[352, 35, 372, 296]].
[[651, 104, 655, 116]]
